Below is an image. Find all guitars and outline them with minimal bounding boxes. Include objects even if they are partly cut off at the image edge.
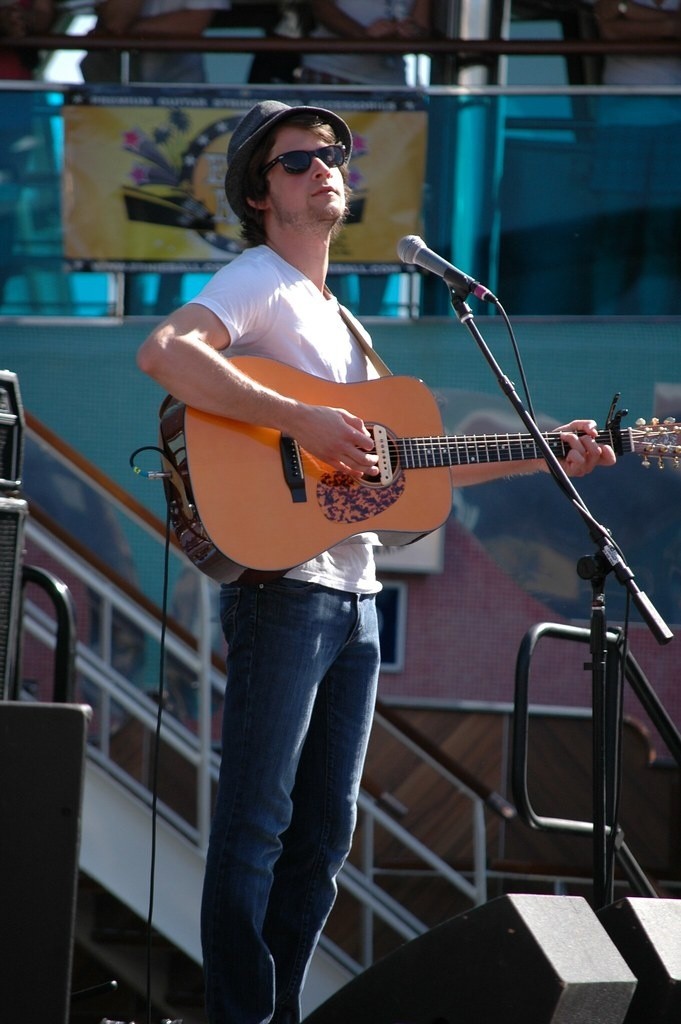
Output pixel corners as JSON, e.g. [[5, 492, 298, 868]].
[[160, 356, 680, 590]]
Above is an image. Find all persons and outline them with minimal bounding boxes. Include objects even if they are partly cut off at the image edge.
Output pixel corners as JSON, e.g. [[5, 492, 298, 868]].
[[0, 0, 681, 317], [133, 99, 617, 1024]]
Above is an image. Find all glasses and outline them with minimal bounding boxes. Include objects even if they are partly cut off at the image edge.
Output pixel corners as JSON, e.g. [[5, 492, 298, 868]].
[[261, 145, 346, 174]]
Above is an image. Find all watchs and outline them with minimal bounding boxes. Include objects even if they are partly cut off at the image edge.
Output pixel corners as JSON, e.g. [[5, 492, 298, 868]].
[[617, 1, 627, 20]]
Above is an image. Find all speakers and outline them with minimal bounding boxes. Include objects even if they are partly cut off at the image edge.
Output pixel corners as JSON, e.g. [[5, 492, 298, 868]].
[[0, 699, 87, 1024], [0, 494, 27, 701], [300, 892, 639, 1024], [594, 895, 681, 1024]]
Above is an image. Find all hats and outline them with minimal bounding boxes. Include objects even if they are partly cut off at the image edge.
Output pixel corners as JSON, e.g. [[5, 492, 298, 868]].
[[225, 100, 353, 222]]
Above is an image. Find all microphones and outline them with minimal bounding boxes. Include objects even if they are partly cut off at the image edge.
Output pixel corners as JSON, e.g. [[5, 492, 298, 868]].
[[397, 234, 499, 305]]
